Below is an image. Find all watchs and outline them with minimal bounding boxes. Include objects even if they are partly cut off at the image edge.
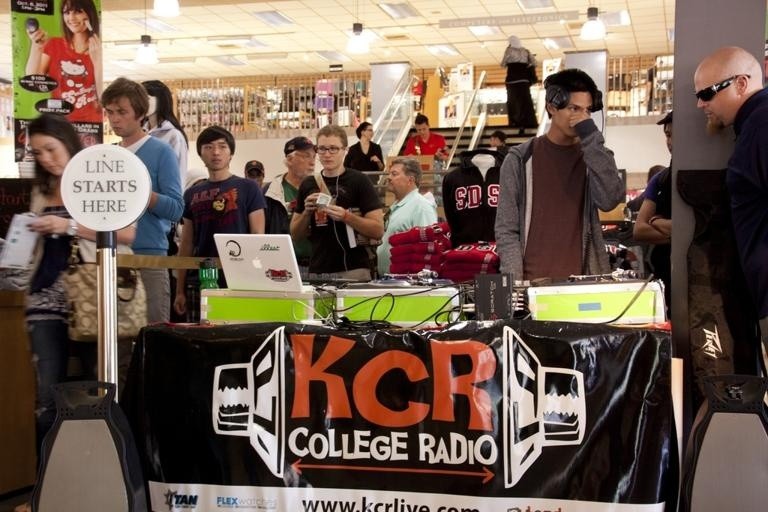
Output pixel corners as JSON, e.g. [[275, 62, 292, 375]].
[[67, 219, 78, 236]]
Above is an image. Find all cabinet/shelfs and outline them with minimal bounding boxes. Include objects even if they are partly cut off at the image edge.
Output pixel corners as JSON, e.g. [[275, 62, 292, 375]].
[[177, 92, 266, 131]]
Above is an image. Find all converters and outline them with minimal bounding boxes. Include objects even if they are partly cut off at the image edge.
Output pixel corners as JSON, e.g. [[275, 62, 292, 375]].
[[475, 272, 513, 319]]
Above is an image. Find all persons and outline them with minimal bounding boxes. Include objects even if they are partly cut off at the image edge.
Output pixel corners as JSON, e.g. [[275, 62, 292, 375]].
[[0, 112, 137, 512], [140, 80, 190, 322], [493, 67, 626, 284], [500, 34, 539, 136], [489, 130, 510, 158], [441, 146, 505, 247], [693, 45, 768, 424], [343, 120, 386, 185], [174, 125, 269, 324], [100, 76, 185, 324], [623, 164, 667, 280], [631, 110, 673, 322], [262, 136, 318, 281], [244, 159, 289, 234], [288, 123, 385, 286], [403, 112, 450, 192], [374, 154, 439, 277], [23, 1, 103, 123]]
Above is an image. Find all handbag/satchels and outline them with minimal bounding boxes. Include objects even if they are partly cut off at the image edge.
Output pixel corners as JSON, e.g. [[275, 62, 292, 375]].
[[365, 246, 377, 268], [527, 64, 537, 83], [67, 263, 147, 343], [433, 161, 446, 183]]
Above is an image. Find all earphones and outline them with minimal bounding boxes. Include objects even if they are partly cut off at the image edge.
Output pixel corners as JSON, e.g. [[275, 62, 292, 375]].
[[345, 151, 348, 156]]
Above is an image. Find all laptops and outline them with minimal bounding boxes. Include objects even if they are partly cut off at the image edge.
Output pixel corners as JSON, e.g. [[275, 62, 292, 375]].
[[213, 232, 313, 293]]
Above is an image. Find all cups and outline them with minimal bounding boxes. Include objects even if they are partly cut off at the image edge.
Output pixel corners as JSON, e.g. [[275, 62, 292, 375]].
[[312, 192, 332, 227]]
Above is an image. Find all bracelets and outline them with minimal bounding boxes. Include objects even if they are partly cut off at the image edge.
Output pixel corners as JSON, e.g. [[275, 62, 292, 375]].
[[649, 216, 661, 225], [344, 209, 351, 225]]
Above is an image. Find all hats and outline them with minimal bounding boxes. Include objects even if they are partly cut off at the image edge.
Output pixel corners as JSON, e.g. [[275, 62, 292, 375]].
[[285, 137, 318, 157], [656, 111, 672, 123], [495, 131, 507, 141], [246, 161, 264, 174]]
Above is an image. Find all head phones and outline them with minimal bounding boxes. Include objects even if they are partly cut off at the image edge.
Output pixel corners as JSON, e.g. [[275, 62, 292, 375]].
[[544, 74, 603, 112]]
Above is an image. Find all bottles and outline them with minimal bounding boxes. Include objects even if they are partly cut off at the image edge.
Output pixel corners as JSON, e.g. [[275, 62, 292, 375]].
[[199, 257, 219, 290]]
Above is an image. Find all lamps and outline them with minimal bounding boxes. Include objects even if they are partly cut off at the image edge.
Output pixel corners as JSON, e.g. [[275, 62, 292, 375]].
[[578, 1, 605, 41], [347, 0, 370, 58], [136, 0, 159, 66]]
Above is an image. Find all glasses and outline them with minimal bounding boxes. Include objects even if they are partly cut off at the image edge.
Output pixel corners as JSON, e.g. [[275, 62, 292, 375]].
[[384, 209, 391, 232], [365, 129, 372, 131], [317, 147, 343, 154], [696, 75, 750, 101]]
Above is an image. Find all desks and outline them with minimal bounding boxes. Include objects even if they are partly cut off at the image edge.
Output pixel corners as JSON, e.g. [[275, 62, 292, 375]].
[[134, 321, 673, 512]]
